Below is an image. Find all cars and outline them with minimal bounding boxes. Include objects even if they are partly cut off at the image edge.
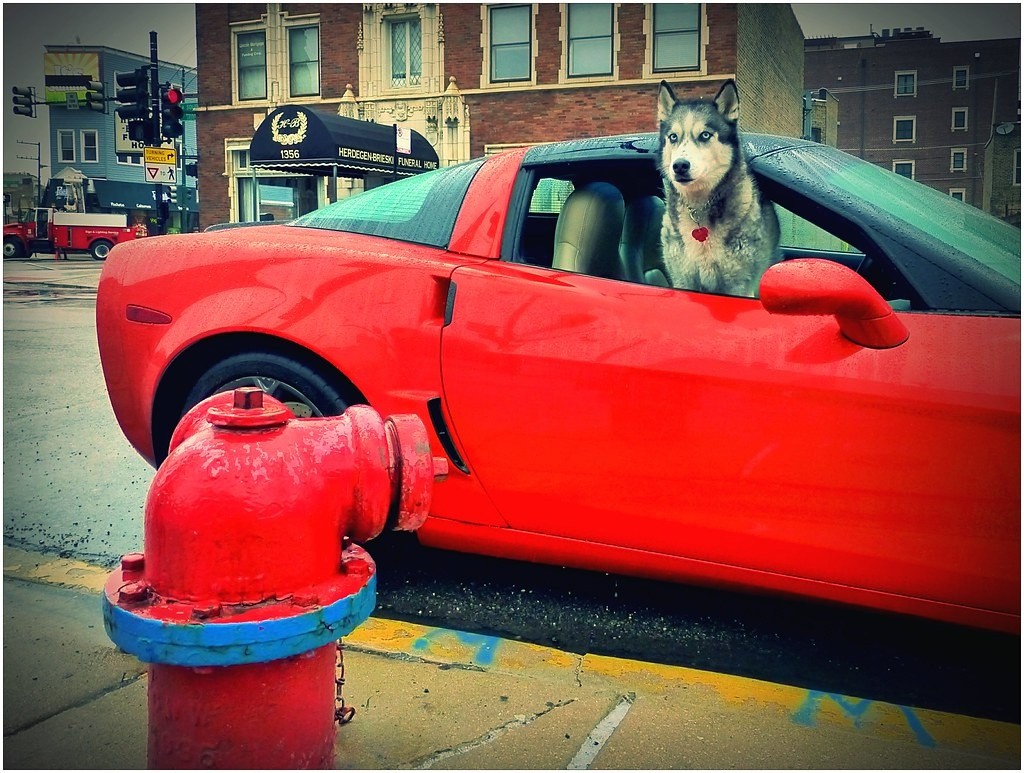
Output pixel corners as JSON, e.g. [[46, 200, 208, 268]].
[[99, 131, 1022, 644]]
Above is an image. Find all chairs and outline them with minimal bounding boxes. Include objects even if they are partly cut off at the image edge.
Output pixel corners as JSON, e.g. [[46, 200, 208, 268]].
[[553, 181, 673, 289]]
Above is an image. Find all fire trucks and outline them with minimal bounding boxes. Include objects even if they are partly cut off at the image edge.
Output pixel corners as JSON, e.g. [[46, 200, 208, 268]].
[[4, 207, 136, 261]]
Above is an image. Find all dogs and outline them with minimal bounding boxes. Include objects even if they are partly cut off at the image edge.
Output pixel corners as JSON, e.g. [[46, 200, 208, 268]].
[[656, 78, 786, 300]]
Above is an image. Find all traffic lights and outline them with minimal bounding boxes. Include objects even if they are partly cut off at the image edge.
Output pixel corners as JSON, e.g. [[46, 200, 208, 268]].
[[116, 66, 149, 121], [86, 80, 109, 114], [167, 185, 182, 208], [129, 121, 153, 143], [12, 85, 38, 119], [159, 87, 186, 140]]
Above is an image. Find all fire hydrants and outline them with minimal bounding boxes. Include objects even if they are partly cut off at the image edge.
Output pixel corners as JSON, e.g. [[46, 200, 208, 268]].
[[97, 385, 451, 769]]
